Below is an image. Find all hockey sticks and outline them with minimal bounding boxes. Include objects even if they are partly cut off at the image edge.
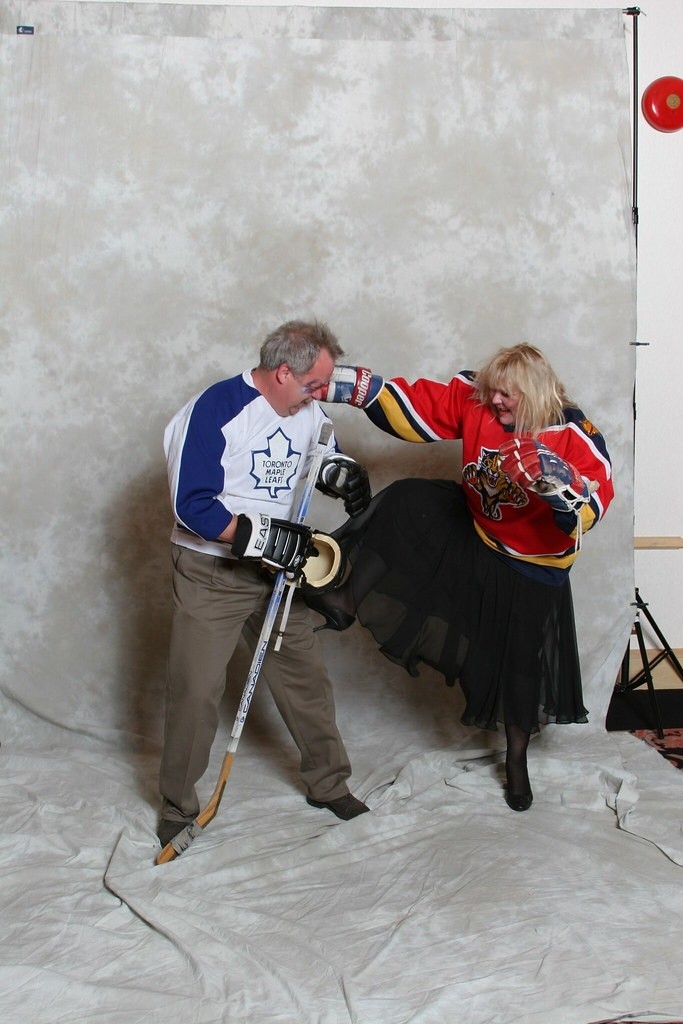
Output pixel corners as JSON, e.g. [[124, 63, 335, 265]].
[[154, 422, 336, 865]]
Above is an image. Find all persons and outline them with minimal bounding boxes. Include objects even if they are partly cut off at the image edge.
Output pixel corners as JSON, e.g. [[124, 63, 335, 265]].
[[155, 321, 372, 850], [313, 344, 614, 812]]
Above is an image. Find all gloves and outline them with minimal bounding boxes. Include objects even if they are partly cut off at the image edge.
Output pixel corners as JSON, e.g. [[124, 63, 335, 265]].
[[231, 512, 319, 580], [321, 365, 384, 408], [314, 457, 371, 518], [498, 439, 592, 554]]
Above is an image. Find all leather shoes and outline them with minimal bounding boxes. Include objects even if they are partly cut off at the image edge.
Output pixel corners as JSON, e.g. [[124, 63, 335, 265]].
[[158, 818, 191, 849], [306, 794, 370, 821]]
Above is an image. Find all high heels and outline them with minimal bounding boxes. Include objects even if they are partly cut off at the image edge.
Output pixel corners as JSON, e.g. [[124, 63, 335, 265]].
[[507, 773, 533, 812], [299, 581, 356, 632]]
